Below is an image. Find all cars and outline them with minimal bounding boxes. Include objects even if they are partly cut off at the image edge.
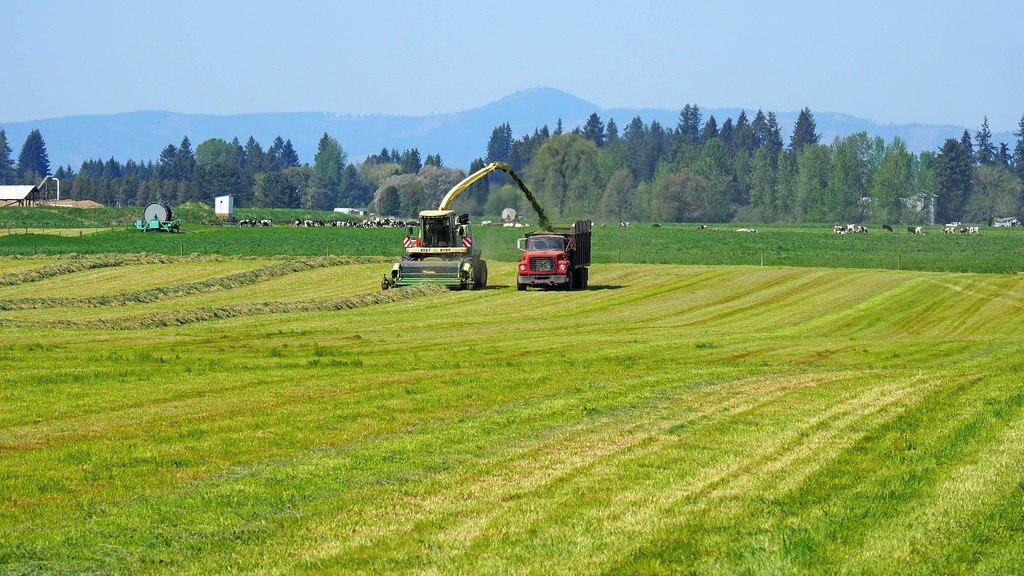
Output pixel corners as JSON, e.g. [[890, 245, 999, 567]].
[[995, 217, 1020, 227], [946, 222, 959, 227]]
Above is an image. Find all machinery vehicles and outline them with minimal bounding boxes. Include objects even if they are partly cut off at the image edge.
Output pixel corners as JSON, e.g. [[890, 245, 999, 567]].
[[381, 162, 512, 290]]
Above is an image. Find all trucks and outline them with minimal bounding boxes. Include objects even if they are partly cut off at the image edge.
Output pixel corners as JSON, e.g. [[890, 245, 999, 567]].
[[516, 219, 592, 291]]
